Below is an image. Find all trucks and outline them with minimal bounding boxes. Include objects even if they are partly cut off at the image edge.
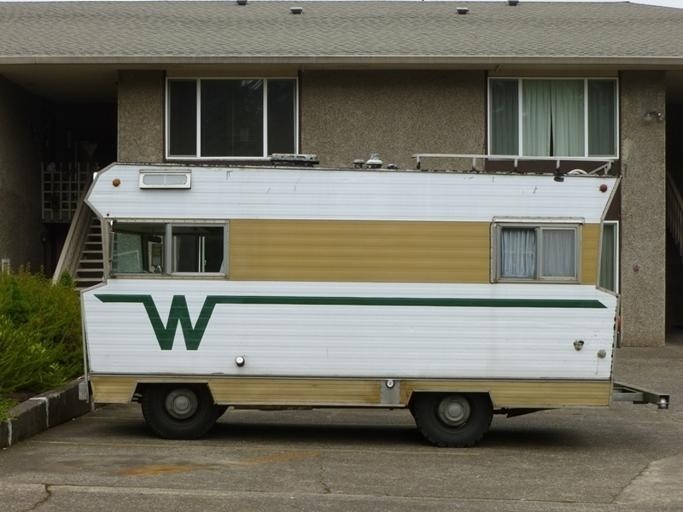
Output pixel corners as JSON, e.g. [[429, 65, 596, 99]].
[[76, 154, 669, 448]]
